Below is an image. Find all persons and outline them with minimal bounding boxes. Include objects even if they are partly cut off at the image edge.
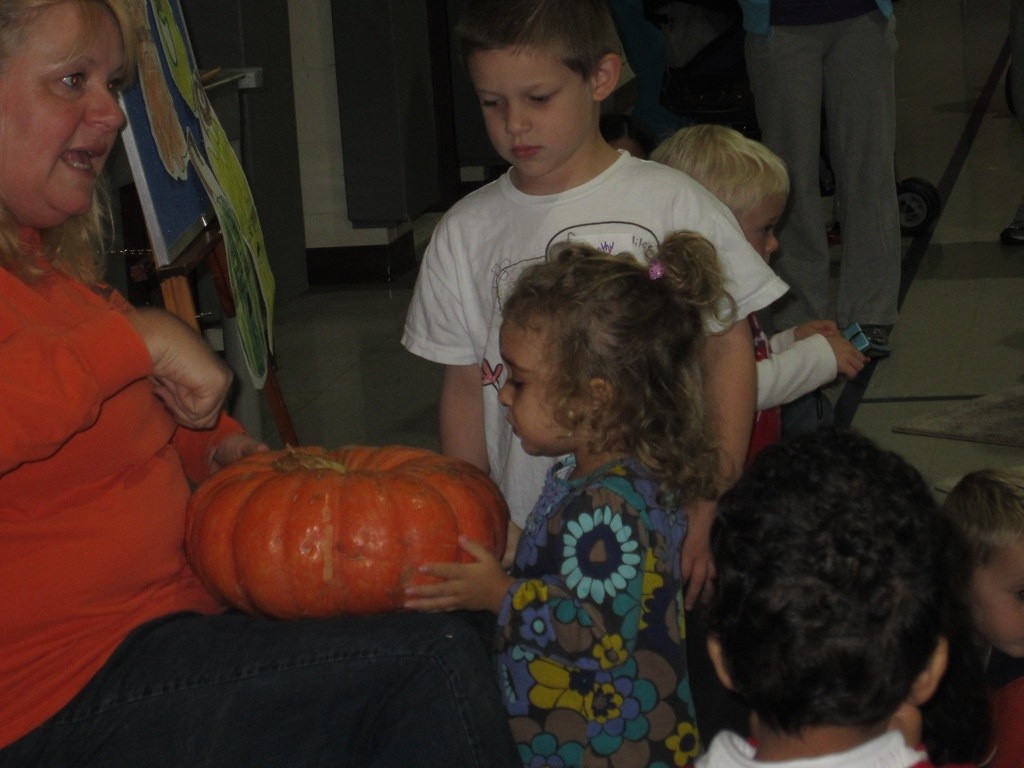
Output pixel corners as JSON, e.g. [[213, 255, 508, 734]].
[[0, 0, 526, 768], [678, 429, 975, 768], [919, 467, 1024, 768], [598, 0, 900, 358], [404, 234, 736, 768], [649, 125, 872, 468], [400, 1, 790, 610]]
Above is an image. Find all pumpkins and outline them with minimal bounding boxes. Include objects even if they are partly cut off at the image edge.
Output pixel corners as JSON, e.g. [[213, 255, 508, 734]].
[[181, 445, 510, 615]]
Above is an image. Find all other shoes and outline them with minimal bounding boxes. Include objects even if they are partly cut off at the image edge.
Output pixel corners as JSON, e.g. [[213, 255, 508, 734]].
[[859, 325, 891, 358], [1000, 201, 1024, 246]]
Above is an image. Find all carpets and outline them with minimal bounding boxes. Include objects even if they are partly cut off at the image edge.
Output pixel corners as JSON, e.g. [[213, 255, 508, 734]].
[[892, 384, 1024, 447]]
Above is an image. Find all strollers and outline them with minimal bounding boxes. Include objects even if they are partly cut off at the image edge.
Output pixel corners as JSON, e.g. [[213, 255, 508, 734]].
[[644, 0, 942, 239]]
